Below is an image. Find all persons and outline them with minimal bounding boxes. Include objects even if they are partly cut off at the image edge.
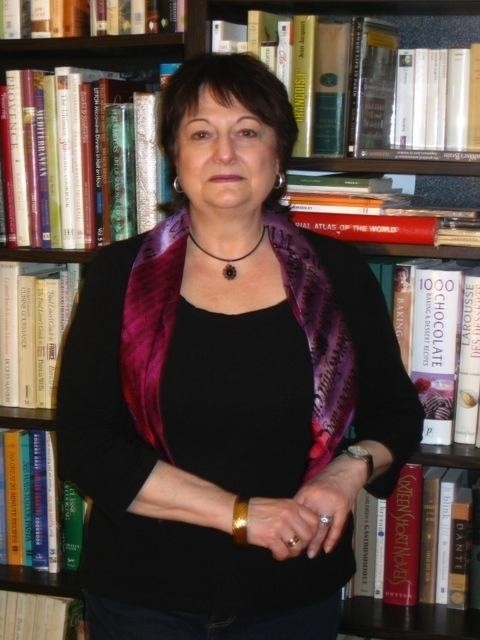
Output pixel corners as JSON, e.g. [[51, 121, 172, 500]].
[[276, 170, 479, 247], [1, 0, 206, 252], [56, 52, 426, 639]]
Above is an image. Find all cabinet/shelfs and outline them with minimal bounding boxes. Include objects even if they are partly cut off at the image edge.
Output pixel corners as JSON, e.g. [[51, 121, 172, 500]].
[[0, 0, 478, 640]]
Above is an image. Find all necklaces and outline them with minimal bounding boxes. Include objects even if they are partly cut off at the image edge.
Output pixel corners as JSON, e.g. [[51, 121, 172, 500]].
[[188, 225, 266, 279]]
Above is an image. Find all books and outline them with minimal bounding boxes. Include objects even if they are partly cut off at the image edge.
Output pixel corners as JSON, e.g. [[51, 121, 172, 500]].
[[368, 260, 479, 445], [342, 463, 475, 610], [1, 427, 90, 638], [206, 10, 479, 158], [0, 261, 84, 409]]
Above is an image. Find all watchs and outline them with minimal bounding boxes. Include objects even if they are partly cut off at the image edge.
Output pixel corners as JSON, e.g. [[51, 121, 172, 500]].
[[340, 444, 374, 483]]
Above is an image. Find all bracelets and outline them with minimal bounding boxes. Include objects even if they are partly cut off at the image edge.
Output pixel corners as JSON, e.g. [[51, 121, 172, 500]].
[[233, 495, 250, 545]]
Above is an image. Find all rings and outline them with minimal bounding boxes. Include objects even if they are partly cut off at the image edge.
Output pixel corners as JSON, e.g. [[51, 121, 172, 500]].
[[286, 536, 299, 547], [321, 514, 333, 523]]
